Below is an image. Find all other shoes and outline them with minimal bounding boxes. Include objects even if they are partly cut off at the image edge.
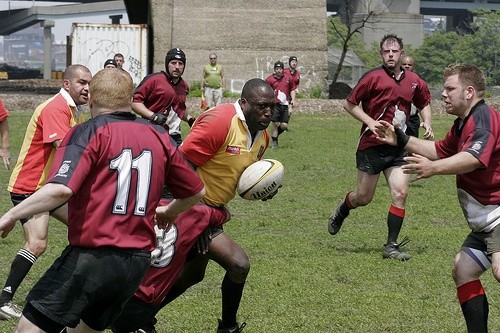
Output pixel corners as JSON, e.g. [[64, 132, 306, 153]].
[[272, 141, 279, 148]]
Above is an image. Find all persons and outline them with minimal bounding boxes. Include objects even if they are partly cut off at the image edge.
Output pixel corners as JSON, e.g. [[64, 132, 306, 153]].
[[264, 60, 293, 149], [372, 62, 500, 333], [200, 53, 225, 112], [0, 68, 207, 333], [0, 64, 92, 321], [401, 57, 420, 156], [107, 77, 282, 333], [282, 56, 301, 132], [328, 34, 435, 262], [113, 53, 127, 73], [104, 59, 117, 69], [0, 98, 12, 172], [130, 48, 198, 147]]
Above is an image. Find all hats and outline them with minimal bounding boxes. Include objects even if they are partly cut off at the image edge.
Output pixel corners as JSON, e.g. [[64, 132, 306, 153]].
[[166, 48, 186, 78], [274, 61, 285, 70], [289, 56, 297, 63], [104, 59, 117, 68]]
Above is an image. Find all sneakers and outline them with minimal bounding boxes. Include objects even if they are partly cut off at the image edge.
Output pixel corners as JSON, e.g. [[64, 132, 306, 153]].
[[217, 319, 247, 333], [0, 304, 23, 321], [382, 236, 412, 260], [328, 200, 349, 235]]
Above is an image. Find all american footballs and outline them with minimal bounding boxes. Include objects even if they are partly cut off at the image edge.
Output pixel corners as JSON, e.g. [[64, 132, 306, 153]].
[[236, 158, 284, 201]]
